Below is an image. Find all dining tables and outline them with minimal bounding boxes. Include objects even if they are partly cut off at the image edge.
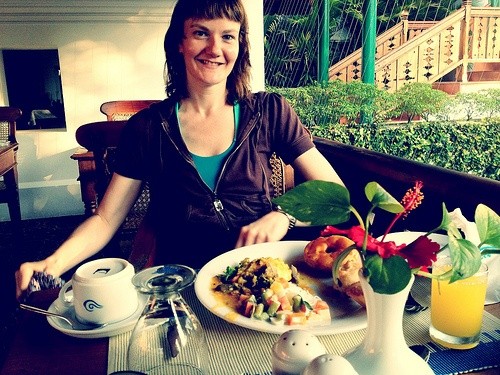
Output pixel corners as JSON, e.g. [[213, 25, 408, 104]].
[[0, 140, 22, 221], [1, 266, 499, 374], [71, 152, 97, 218]]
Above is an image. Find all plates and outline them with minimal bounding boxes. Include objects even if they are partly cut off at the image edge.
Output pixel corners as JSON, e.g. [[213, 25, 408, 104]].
[[46, 288, 150, 338], [194, 240, 367, 336], [375, 231, 449, 278]]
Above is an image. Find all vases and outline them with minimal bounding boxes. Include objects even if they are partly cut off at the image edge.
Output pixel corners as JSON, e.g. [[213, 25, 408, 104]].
[[341, 267, 433, 374]]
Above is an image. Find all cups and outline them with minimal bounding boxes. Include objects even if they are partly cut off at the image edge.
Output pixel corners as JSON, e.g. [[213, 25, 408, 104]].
[[127, 264, 212, 375], [58, 257, 139, 325], [429, 258, 489, 350]]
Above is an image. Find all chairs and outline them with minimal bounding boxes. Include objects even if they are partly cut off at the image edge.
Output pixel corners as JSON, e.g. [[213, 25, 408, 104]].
[[76, 121, 186, 281], [0, 105, 22, 188], [100, 100, 162, 121]]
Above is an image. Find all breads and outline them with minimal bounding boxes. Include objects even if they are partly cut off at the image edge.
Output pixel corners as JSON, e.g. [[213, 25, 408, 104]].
[[331, 247, 365, 308]]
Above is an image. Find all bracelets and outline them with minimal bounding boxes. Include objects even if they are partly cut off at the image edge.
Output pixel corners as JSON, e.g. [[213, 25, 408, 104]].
[[274, 205, 297, 230]]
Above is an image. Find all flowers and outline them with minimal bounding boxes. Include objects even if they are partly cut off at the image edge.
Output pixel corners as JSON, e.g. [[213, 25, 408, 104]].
[[280, 176, 499, 294]]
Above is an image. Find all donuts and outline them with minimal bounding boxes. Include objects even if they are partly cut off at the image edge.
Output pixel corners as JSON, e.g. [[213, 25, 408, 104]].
[[304, 235, 355, 271]]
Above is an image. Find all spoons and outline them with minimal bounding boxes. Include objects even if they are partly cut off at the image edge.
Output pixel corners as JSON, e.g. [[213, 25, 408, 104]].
[[18, 304, 108, 330]]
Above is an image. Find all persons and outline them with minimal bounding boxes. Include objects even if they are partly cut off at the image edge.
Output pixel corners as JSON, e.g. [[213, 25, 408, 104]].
[[15, 0, 348, 311]]
